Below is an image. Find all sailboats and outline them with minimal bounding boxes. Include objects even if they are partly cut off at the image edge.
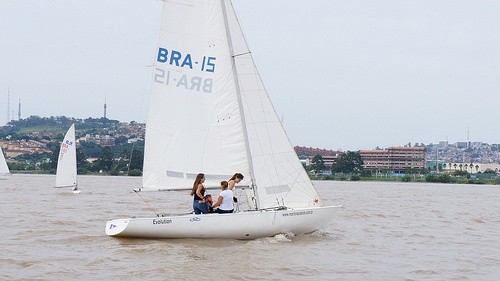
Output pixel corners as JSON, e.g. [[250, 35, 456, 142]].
[[104, 0, 344, 240], [52, 122, 80, 194], [0, 147, 11, 180]]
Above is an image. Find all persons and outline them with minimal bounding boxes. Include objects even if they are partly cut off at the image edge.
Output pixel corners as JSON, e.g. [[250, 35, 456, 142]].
[[227, 173, 244, 193], [191, 173, 209, 215], [211, 181, 234, 213]]
